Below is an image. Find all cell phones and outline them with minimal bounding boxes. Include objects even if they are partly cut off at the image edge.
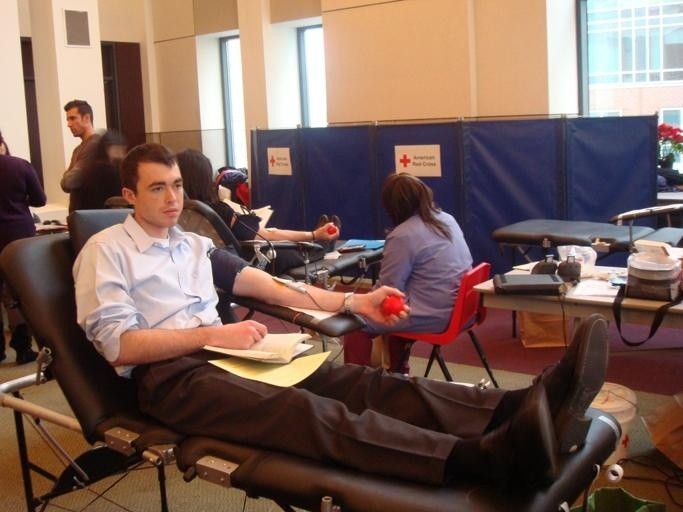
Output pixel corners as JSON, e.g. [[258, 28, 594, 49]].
[[338, 245, 366, 252]]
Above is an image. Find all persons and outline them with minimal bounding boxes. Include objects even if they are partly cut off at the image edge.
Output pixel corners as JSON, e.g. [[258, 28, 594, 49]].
[[60, 100, 105, 224], [170, 149, 340, 245], [70, 142, 611, 488], [70, 130, 130, 208], [0, 133, 48, 363], [353, 172, 476, 340]]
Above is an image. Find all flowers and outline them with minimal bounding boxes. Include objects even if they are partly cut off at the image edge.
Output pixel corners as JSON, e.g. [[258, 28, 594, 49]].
[[657, 124, 683, 176]]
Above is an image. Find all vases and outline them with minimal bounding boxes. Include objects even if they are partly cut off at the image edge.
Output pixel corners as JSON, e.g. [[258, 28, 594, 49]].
[[657, 176, 667, 186]]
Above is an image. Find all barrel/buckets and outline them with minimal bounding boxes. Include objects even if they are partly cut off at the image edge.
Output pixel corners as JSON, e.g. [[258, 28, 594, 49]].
[[583, 376, 641, 467]]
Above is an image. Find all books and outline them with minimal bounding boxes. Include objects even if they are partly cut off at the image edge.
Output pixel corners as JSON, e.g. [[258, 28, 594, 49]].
[[200, 325, 315, 365]]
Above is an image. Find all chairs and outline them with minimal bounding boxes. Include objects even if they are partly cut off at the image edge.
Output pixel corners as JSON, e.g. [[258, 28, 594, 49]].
[[177, 199, 385, 294], [490, 203, 683, 264], [0, 208, 622, 511], [384, 262, 499, 389]]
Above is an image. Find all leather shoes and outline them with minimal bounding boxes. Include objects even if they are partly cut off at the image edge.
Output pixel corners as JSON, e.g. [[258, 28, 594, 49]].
[[525, 312, 611, 458], [478, 385, 559, 492]]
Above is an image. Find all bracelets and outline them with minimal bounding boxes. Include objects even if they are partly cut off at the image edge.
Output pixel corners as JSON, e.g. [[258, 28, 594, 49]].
[[343, 291, 352, 317], [310, 230, 314, 241]]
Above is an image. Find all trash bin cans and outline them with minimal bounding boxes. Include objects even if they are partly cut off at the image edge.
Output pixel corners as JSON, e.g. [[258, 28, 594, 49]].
[[514, 311, 572, 348]]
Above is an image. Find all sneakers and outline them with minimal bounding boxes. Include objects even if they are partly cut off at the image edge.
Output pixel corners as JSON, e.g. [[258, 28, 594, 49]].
[[311, 212, 342, 253]]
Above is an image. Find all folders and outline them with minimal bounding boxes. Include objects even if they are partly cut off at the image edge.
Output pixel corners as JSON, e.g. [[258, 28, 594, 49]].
[[337, 237, 385, 251]]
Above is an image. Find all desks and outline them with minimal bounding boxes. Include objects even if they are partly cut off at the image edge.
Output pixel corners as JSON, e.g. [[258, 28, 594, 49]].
[[472, 259, 683, 331]]
